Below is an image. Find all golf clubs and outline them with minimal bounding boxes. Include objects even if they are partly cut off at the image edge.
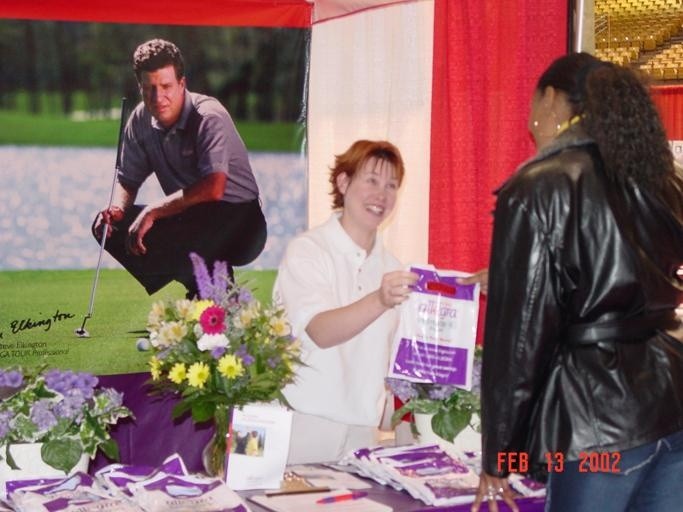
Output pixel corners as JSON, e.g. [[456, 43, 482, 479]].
[[75, 97, 127, 338]]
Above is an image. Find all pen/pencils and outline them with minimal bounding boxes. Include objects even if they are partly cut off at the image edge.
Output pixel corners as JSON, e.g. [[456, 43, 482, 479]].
[[528, 499, 545, 503], [316, 492, 368, 503]]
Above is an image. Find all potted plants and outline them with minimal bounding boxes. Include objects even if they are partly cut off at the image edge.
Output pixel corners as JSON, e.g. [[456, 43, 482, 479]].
[[391, 384, 480, 450]]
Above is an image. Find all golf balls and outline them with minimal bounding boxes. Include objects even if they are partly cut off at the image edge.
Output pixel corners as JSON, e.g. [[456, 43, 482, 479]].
[[138, 338, 149, 352]]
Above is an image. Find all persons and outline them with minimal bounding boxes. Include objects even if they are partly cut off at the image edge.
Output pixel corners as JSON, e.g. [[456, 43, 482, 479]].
[[270, 139, 490, 466], [468, 51, 682, 512], [90, 38, 267, 353]]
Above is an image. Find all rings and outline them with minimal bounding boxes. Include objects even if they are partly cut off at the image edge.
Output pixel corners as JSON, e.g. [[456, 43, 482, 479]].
[[497, 487, 505, 493], [487, 485, 495, 501]]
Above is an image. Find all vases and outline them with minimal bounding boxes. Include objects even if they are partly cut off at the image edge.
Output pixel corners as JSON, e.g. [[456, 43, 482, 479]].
[[0, 443, 92, 512], [202, 402, 232, 479]]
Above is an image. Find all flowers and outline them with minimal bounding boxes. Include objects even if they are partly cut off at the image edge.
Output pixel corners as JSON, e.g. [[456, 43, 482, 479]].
[[0, 365, 127, 443], [138, 255, 299, 404]]
